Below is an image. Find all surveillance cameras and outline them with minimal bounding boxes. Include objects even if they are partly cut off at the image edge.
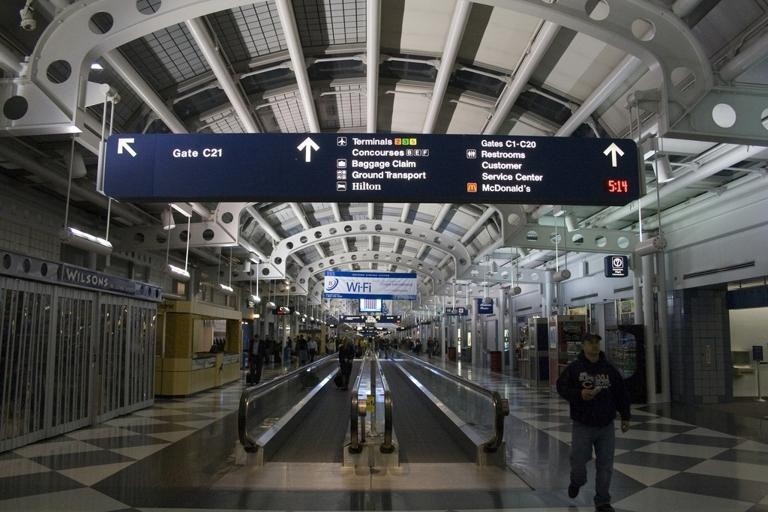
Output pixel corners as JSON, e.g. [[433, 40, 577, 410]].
[[21, 20, 36, 31]]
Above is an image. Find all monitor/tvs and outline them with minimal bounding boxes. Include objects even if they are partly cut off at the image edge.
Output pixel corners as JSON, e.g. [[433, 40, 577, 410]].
[[731, 351, 749, 363], [210, 345, 216, 353]]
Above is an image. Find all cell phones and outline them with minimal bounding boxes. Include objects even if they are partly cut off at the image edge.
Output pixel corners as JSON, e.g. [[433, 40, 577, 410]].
[[591, 386, 602, 396]]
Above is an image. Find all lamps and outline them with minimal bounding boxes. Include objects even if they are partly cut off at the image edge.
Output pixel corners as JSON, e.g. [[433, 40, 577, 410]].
[[248, 264, 335, 327], [644, 154, 674, 184], [243, 253, 251, 273], [166, 202, 191, 283], [161, 202, 175, 230], [248, 252, 260, 264], [58, 77, 115, 256], [450, 198, 667, 314], [217, 246, 234, 296]]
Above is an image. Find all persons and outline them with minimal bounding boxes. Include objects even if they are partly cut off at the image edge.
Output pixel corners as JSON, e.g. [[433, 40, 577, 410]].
[[337, 336, 354, 391], [260, 330, 440, 369], [246, 333, 269, 387], [555, 331, 633, 511]]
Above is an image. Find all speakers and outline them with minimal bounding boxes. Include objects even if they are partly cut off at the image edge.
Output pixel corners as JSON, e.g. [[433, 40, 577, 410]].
[[753, 345, 763, 361]]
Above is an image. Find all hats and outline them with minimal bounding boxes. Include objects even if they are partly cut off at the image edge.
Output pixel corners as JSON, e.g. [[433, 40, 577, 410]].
[[583, 332, 602, 340]]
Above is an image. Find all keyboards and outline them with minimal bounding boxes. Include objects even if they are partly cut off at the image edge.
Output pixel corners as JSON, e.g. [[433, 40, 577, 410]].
[[732, 366, 750, 369]]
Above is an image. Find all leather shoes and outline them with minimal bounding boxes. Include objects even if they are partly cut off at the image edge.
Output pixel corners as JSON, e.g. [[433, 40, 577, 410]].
[[596, 503, 616, 512], [568, 481, 580, 499]]
